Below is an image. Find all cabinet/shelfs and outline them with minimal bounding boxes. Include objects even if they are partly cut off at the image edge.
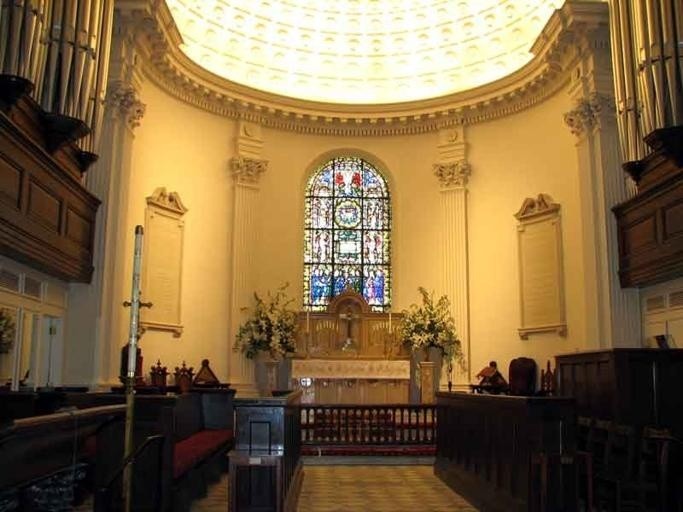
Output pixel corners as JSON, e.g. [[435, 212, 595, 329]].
[[224, 390, 303, 512], [431, 388, 576, 512], [555, 348, 683, 512]]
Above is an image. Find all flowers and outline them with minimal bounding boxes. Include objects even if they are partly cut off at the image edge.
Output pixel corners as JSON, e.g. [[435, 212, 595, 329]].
[[231, 281, 301, 362], [396, 284, 466, 384]]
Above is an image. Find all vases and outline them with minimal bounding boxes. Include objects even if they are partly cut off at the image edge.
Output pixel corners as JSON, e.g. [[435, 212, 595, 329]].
[[254, 348, 289, 395], [410, 346, 444, 392]]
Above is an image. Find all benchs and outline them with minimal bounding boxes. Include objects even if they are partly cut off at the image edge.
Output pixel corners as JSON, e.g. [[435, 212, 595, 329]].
[[165, 428, 233, 512]]
